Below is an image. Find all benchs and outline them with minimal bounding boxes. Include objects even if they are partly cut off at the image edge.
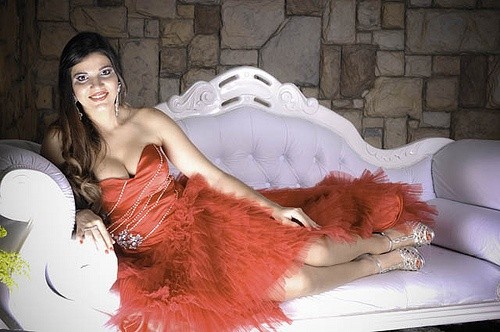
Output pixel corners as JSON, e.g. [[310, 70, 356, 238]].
[[0, 66, 499, 332]]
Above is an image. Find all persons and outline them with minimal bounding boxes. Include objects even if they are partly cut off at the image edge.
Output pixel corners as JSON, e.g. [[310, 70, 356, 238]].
[[37, 31, 437, 331]]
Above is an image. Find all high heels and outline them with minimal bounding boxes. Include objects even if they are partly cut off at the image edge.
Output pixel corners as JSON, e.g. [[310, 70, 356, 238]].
[[356, 246, 425, 275], [380, 220, 435, 252]]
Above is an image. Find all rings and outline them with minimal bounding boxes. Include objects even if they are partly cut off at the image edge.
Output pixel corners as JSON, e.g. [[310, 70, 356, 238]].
[[91, 225, 97, 230], [84, 227, 91, 231]]
